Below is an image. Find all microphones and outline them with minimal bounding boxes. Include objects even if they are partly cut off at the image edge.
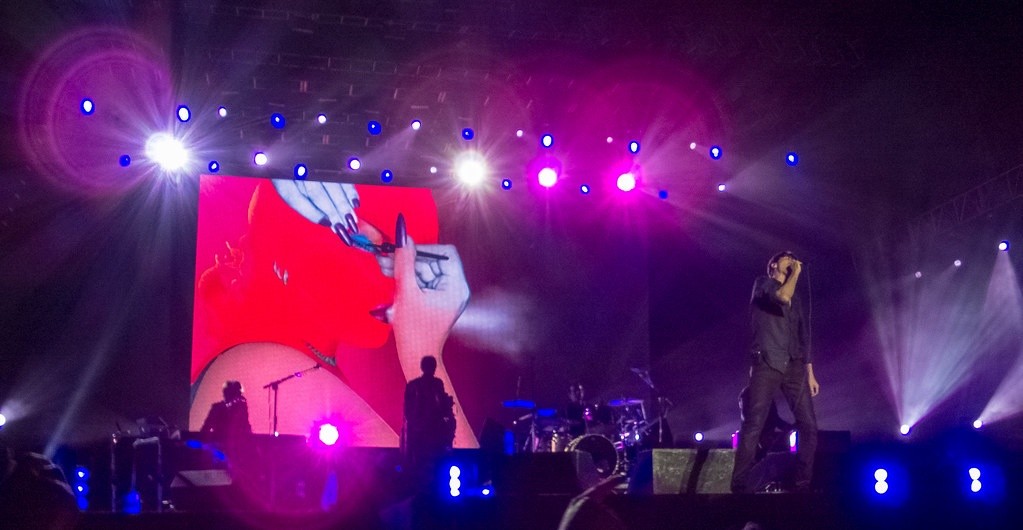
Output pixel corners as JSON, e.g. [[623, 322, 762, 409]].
[[787, 264, 805, 271]]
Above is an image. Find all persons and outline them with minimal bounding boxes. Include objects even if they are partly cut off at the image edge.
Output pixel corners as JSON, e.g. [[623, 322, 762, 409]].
[[188, 178, 484, 448], [556, 382, 595, 441], [403, 355, 457, 447], [727, 250, 818, 495], [200, 380, 252, 433], [0, 427, 87, 530]]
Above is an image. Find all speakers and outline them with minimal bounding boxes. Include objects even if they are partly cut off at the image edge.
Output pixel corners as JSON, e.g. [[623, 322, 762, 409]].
[[499, 450, 600, 495], [626, 449, 737, 493], [170, 469, 241, 512]]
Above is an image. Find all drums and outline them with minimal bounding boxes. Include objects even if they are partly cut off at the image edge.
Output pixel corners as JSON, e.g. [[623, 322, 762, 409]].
[[564, 434, 619, 485]]
